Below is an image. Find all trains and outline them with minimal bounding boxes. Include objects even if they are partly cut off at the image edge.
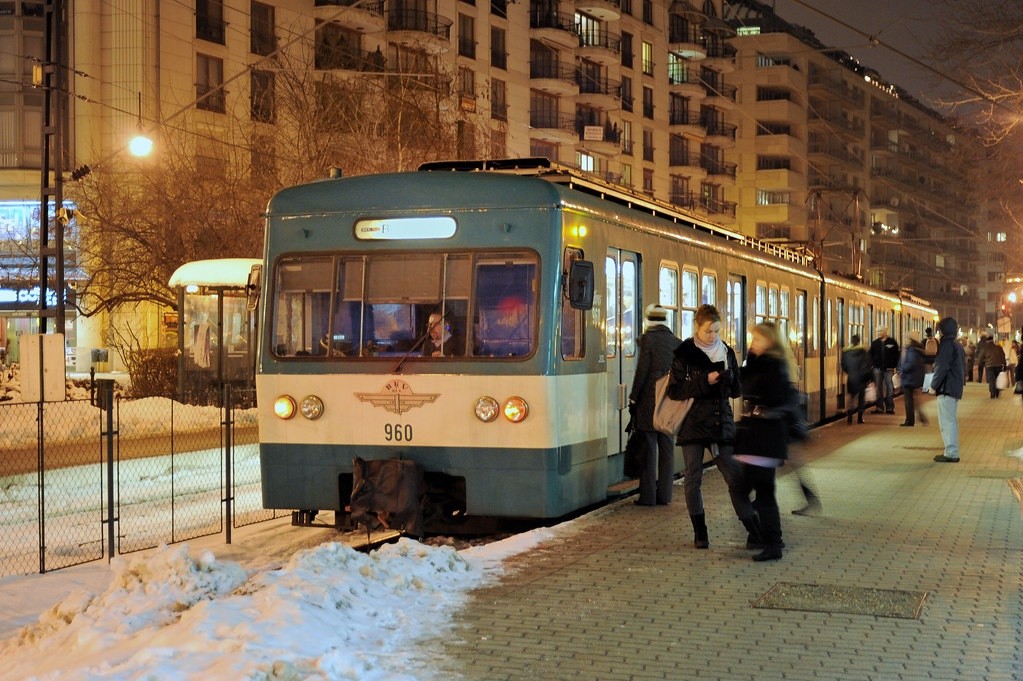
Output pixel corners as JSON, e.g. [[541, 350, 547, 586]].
[[244, 156, 941, 534]]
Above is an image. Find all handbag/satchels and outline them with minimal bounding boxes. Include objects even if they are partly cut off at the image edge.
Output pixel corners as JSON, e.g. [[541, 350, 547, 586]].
[[892, 368, 901, 388], [653, 365, 694, 438], [865, 381, 877, 402], [732, 405, 787, 459], [624, 415, 647, 477], [995, 370, 1008, 390]]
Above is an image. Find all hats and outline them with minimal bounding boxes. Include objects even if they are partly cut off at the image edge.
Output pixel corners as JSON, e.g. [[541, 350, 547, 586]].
[[909, 331, 920, 342], [939, 317, 958, 335], [646, 303, 667, 321]]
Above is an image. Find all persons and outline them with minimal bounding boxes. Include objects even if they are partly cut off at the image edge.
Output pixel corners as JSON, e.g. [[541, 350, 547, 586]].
[[728, 322, 823, 562], [929, 317, 965, 463], [869, 325, 900, 414], [668, 304, 786, 550], [841, 335, 867, 425], [473, 297, 574, 359], [422, 306, 479, 356], [628, 303, 684, 507], [956, 334, 1023, 399], [897, 328, 942, 427]]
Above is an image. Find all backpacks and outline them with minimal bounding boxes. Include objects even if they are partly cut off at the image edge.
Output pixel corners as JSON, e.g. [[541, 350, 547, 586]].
[[924, 337, 937, 355]]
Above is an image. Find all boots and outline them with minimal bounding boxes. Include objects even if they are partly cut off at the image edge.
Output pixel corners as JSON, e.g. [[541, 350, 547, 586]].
[[740, 514, 785, 550], [689, 510, 709, 548], [753, 505, 782, 561], [847, 410, 854, 423], [858, 412, 864, 424]]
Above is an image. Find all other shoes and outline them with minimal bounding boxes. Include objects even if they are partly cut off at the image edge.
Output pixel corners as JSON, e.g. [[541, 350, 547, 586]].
[[991, 389, 999, 399], [886, 410, 895, 414], [634, 499, 668, 507], [871, 410, 884, 415], [899, 423, 914, 426], [933, 454, 960, 463]]
